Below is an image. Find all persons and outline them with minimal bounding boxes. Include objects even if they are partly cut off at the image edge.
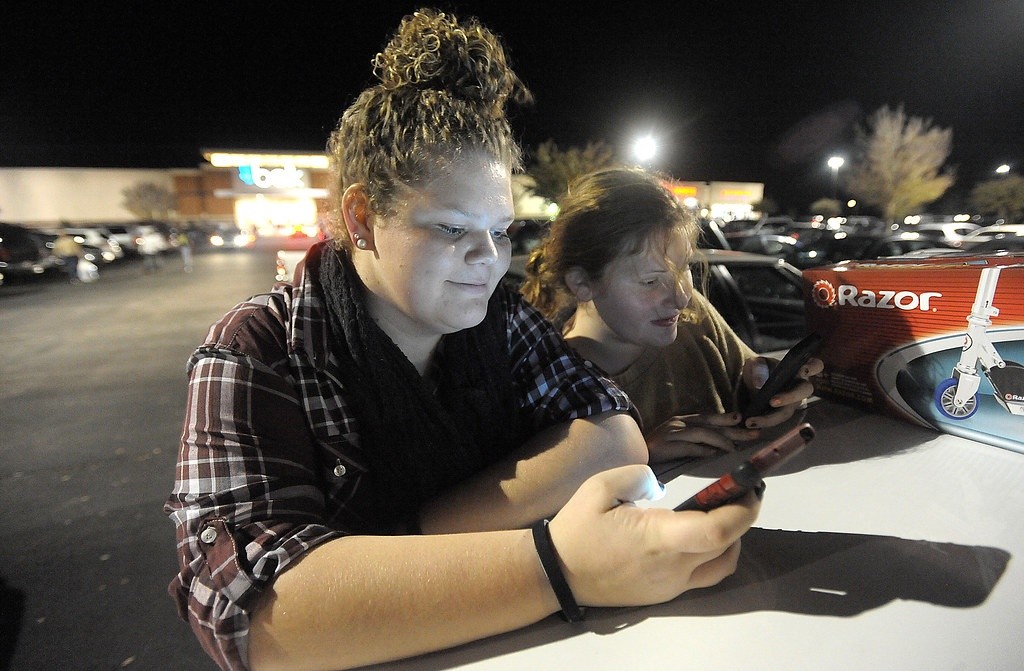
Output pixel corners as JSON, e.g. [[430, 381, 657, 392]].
[[522, 168, 824, 464], [138, 226, 192, 274], [165, 9, 765, 671], [52, 227, 85, 284]]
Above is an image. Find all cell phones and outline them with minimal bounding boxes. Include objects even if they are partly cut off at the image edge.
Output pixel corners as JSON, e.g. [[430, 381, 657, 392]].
[[673, 423, 815, 512], [741, 333, 822, 428]]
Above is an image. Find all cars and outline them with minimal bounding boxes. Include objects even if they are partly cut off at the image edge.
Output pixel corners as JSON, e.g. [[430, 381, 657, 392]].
[[505, 213, 556, 279], [0, 215, 257, 290], [690, 212, 1024, 354]]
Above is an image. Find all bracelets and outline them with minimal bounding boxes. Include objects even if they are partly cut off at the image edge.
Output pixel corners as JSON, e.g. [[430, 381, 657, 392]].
[[531, 519, 588, 624]]
